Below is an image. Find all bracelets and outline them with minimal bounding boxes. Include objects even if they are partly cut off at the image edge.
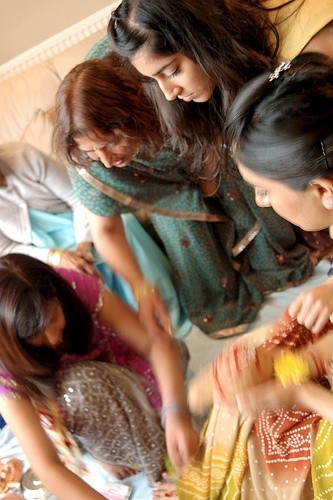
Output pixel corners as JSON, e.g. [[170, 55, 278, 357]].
[[131, 282, 155, 299], [157, 401, 191, 425], [303, 344, 328, 383]]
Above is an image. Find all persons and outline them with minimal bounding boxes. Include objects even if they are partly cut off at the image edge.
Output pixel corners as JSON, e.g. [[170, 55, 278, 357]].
[[0, 252, 205, 500], [0, 140, 194, 340], [173, 52, 333, 500], [107, 0, 333, 333], [51, 33, 314, 339]]
